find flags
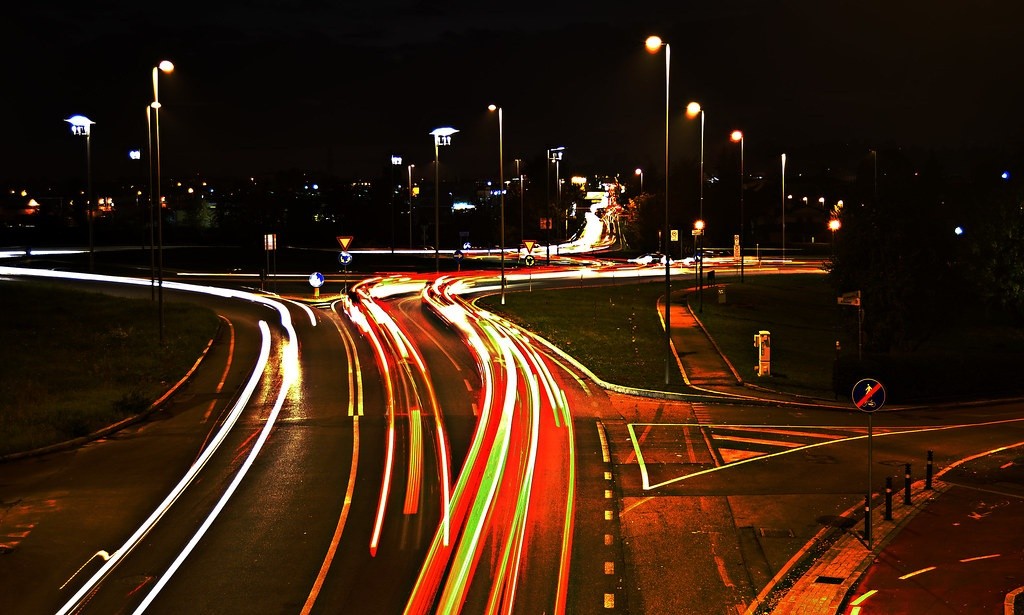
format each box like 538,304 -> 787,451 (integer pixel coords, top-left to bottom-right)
838,290 -> 859,306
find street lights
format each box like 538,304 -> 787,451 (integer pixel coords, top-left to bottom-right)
152,60 -> 174,347
62,114 -> 96,272
686,101 -> 704,284
644,34 -> 670,340
428,127 -> 461,274
781,151 -> 786,263
729,130 -> 745,282
635,168 -> 643,192
146,101 -> 162,302
487,104 -> 505,305
408,164 -> 416,249
